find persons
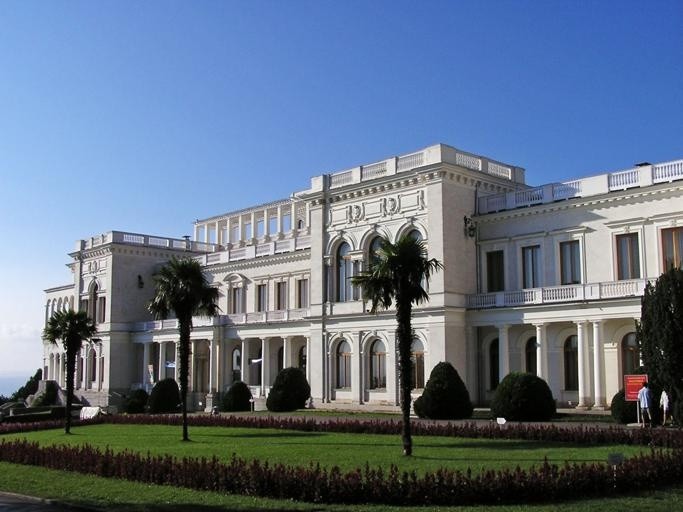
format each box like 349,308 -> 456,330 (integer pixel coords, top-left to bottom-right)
659,385 -> 674,427
637,381 -> 652,428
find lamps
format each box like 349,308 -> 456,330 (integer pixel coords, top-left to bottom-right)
464,216 -> 476,237
137,275 -> 144,289
